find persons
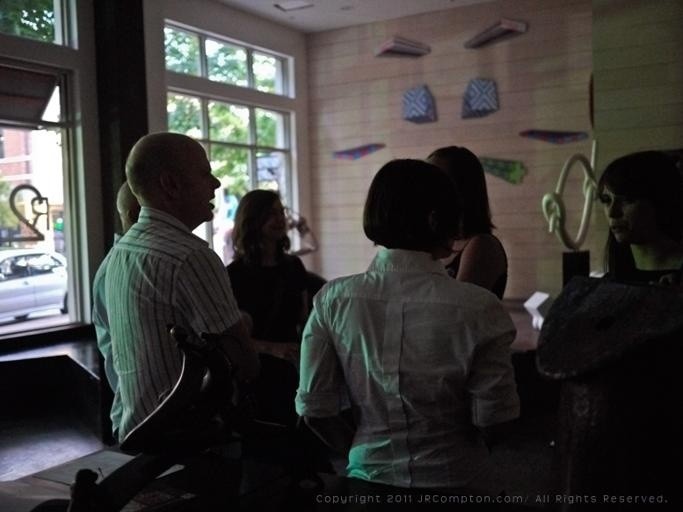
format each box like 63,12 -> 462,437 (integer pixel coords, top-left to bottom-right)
293,161 -> 521,512
92,180 -> 140,445
427,146 -> 508,301
541,151 -> 682,512
225,190 -> 309,361
91,133 -> 259,448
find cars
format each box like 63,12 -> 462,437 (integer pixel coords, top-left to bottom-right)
0,248 -> 68,321
